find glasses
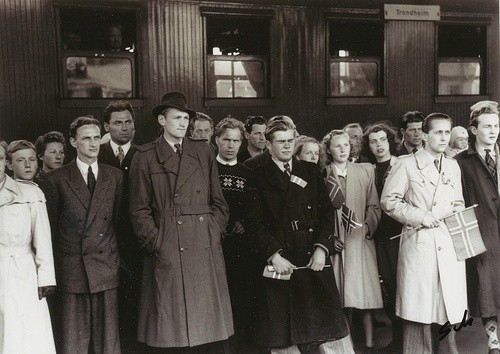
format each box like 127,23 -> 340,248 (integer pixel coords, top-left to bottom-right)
219,136 -> 241,145
350,135 -> 363,141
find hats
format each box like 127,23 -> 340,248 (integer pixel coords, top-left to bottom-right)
153,92 -> 196,119
469,100 -> 498,111
450,125 -> 469,148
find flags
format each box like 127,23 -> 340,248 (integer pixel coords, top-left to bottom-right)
327,169 -> 344,208
440,204 -> 487,262
263,265 -> 293,280
341,203 -> 364,235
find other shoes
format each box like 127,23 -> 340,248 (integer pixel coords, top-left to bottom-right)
365,344 -> 376,354
488,349 -> 500,354
379,341 -> 403,353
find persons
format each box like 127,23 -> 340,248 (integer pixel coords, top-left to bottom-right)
0,92 -> 500,354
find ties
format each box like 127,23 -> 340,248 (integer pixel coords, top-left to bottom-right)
412,148 -> 418,154
88,166 -> 97,197
117,146 -> 125,167
174,144 -> 182,160
484,149 -> 498,191
284,163 -> 291,178
434,159 -> 439,174
